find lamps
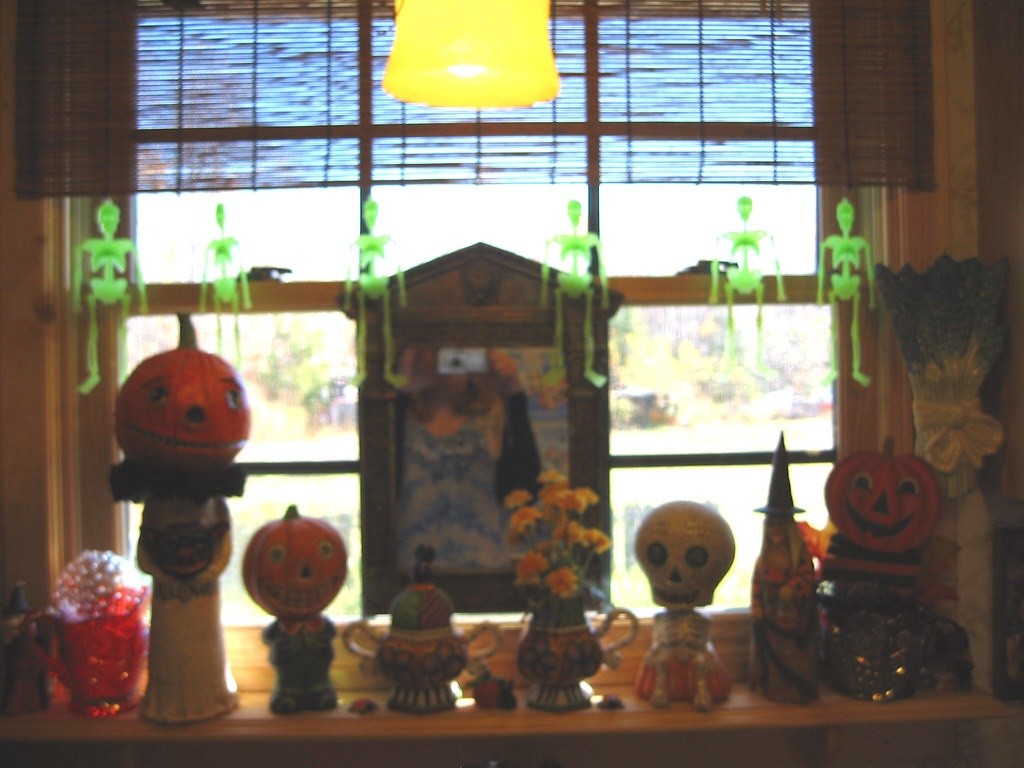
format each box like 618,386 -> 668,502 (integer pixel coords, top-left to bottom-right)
381,0 -> 561,109
